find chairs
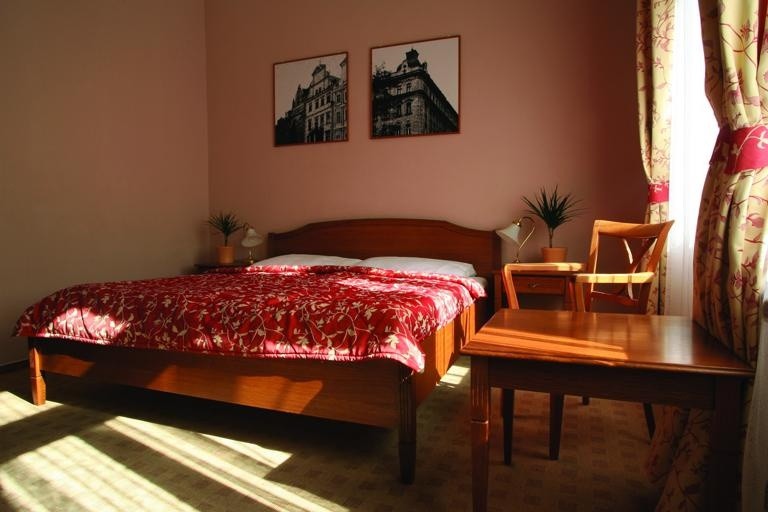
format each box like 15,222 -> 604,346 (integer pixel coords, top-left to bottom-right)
501,216 -> 680,471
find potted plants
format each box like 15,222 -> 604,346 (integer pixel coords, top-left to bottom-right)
201,212 -> 245,263
518,184 -> 586,261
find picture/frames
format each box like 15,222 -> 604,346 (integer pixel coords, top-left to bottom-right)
273,48 -> 350,149
369,34 -> 463,141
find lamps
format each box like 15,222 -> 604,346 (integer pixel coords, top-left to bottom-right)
491,213 -> 537,262
238,218 -> 263,261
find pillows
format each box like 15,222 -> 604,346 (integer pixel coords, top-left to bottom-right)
353,255 -> 478,282
247,253 -> 362,271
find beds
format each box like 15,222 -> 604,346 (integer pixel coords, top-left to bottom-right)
9,216 -> 506,484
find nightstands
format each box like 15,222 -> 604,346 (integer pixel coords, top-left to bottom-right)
488,265 -> 574,311
194,260 -> 252,273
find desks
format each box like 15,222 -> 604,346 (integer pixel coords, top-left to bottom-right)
455,307 -> 756,509
